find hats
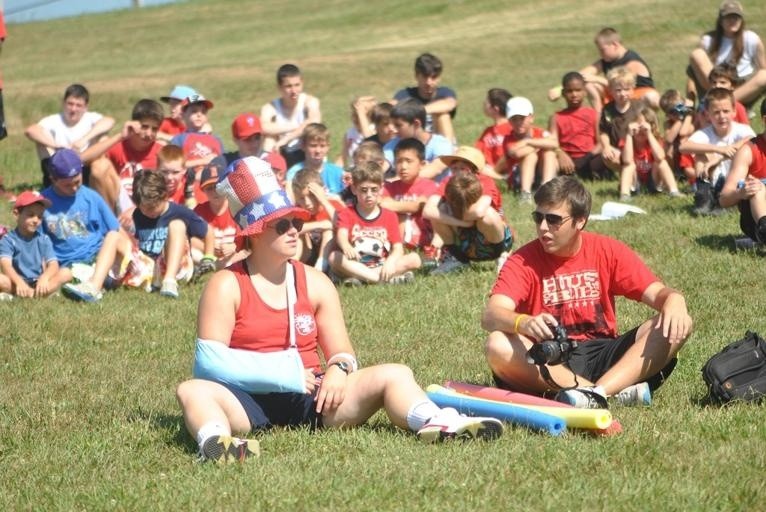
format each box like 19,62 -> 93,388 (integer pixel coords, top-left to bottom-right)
263,154 -> 286,172
201,165 -> 222,188
719,0 -> 744,17
180,94 -> 213,113
49,150 -> 81,178
232,112 -> 262,139
15,190 -> 49,208
505,96 -> 533,119
215,157 -> 310,253
160,85 -> 196,103
439,145 -> 485,171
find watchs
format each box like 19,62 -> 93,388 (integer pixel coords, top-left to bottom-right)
330,356 -> 354,373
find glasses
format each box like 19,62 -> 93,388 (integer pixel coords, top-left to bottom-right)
531,211 -> 570,226
262,219 -> 304,238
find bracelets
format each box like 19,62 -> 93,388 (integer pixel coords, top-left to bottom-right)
511,310 -> 525,334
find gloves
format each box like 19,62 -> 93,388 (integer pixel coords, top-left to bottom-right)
197,258 -> 216,275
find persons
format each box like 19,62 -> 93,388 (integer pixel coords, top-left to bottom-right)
719,100 -> 766,257
474,89 -> 561,205
175,155 -> 502,464
688,0 -> 765,111
390,53 -> 460,143
0,64 -> 517,301
547,28 -> 758,216
479,175 -> 692,410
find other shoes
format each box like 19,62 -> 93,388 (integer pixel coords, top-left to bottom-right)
2,292 -> 14,301
61,281 -> 105,303
390,271 -> 415,284
430,257 -> 470,276
345,277 -> 362,286
48,292 -> 62,299
159,281 -> 179,297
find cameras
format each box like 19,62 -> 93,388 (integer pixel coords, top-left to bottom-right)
524,324 -> 579,366
672,102 -> 690,118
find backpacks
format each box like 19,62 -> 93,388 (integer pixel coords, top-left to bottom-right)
700,329 -> 766,405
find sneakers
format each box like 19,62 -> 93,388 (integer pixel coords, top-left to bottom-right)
558,387 -> 609,408
416,407 -> 504,445
691,173 -> 753,252
614,381 -> 650,408
200,435 -> 260,463
